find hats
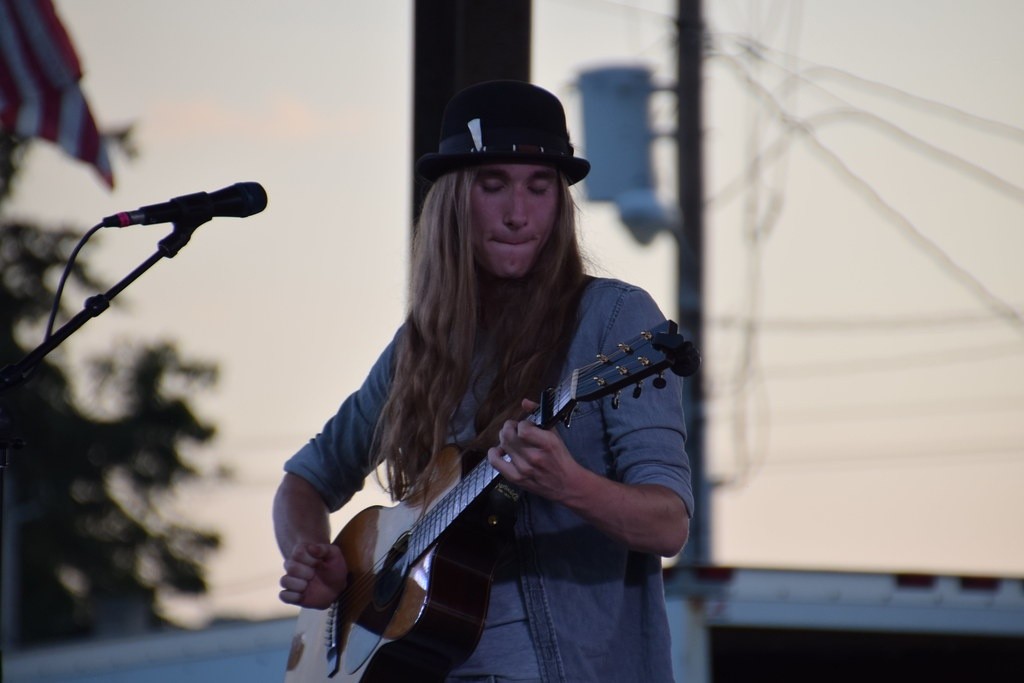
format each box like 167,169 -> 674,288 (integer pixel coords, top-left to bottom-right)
414,79 -> 591,187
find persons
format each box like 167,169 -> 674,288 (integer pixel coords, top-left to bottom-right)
267,81 -> 697,683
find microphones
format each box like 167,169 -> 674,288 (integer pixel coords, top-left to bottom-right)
104,181 -> 267,228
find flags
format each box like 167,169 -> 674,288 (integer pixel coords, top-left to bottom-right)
0,0 -> 119,189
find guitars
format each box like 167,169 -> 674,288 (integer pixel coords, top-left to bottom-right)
282,320 -> 703,683
